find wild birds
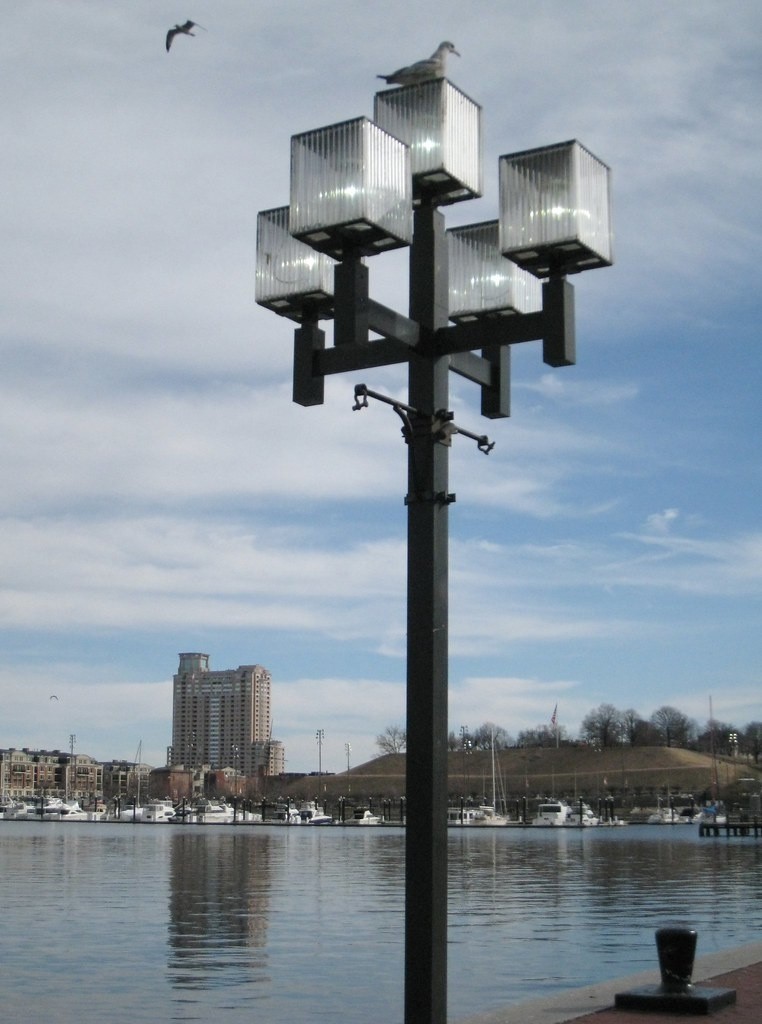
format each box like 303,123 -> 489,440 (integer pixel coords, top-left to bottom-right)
166,20 -> 208,53
49,696 -> 59,700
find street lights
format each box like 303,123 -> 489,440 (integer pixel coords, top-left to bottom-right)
257,77 -> 616,1024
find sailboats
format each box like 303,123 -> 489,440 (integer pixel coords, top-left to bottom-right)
690,695 -> 726,824
469,731 -> 510,827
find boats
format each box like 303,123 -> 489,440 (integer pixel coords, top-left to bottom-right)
448,808 -> 483,825
532,800 -> 600,826
344,808 -> 381,825
0,739 -> 332,826
648,807 -> 680,822
